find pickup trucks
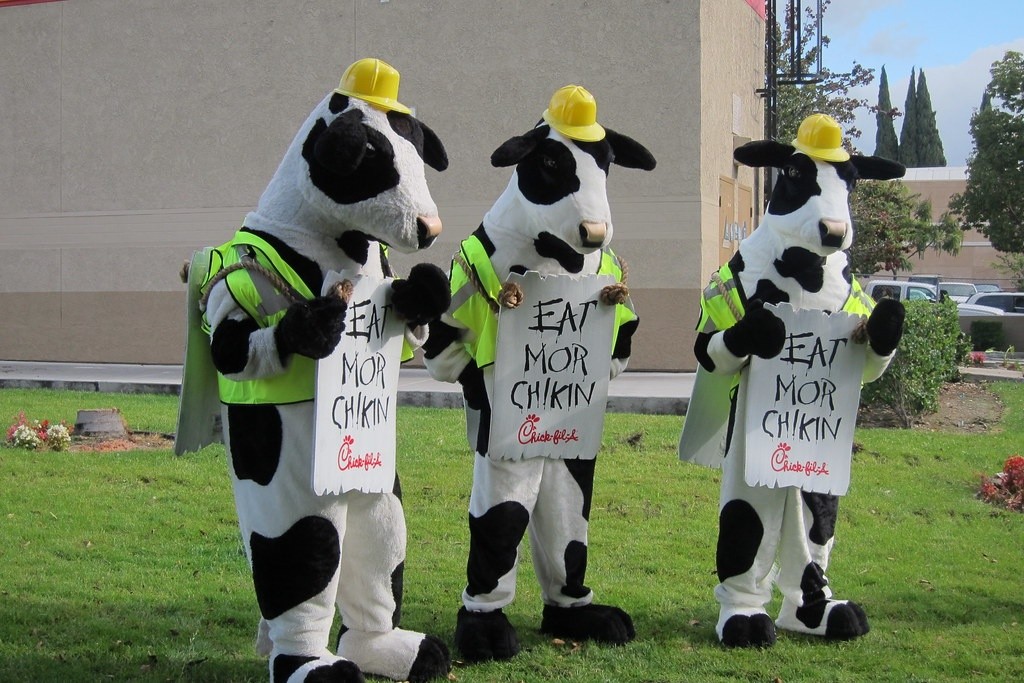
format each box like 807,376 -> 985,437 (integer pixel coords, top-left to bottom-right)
866,281 -> 1003,318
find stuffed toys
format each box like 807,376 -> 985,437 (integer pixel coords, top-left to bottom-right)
201,56 -> 453,682
694,111 -> 906,648
419,82 -> 658,660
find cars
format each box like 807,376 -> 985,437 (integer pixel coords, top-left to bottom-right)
974,283 -> 1003,295
938,282 -> 976,304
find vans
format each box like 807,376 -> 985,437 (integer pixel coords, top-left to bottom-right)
965,292 -> 1024,313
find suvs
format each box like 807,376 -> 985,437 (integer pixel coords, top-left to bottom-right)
909,273 -> 945,287
